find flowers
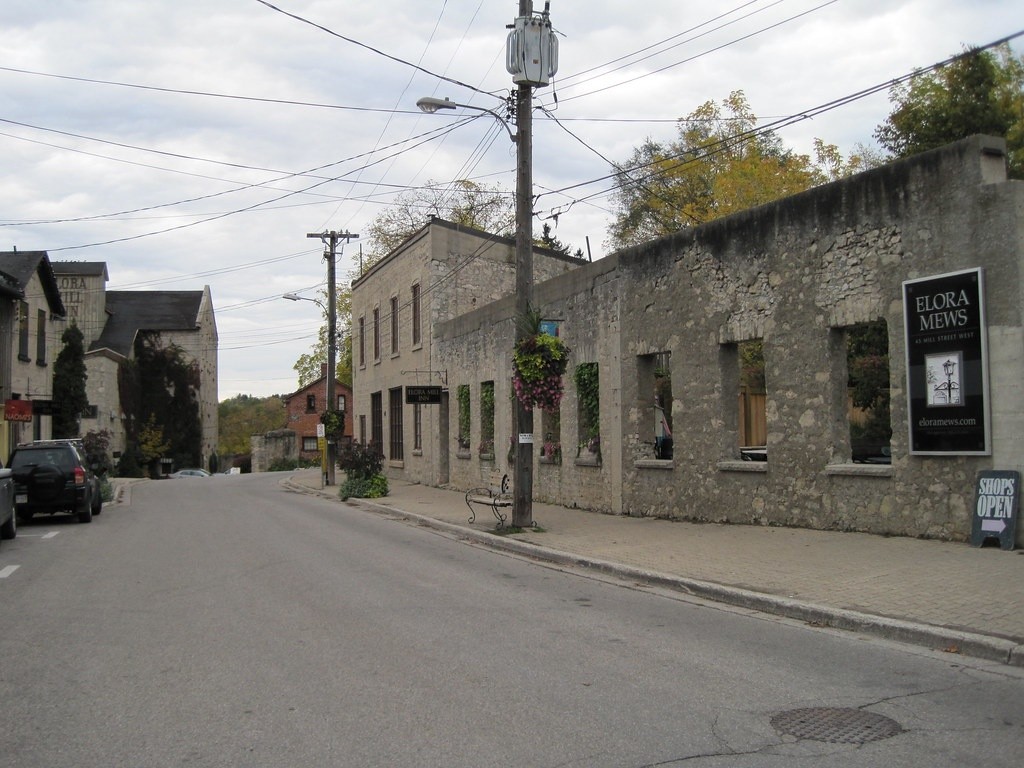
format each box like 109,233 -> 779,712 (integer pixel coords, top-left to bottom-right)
513,372 -> 563,410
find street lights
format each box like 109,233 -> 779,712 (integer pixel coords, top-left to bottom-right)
943,358 -> 957,404
417,96 -> 536,527
283,293 -> 337,487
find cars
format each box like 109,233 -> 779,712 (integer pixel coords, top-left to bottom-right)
172,468 -> 212,477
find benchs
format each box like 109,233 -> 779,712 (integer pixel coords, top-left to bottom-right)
465,468 -> 513,530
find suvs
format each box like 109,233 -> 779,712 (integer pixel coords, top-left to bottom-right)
5,439 -> 101,523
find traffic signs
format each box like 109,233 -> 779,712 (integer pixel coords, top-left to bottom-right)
971,469 -> 1021,551
